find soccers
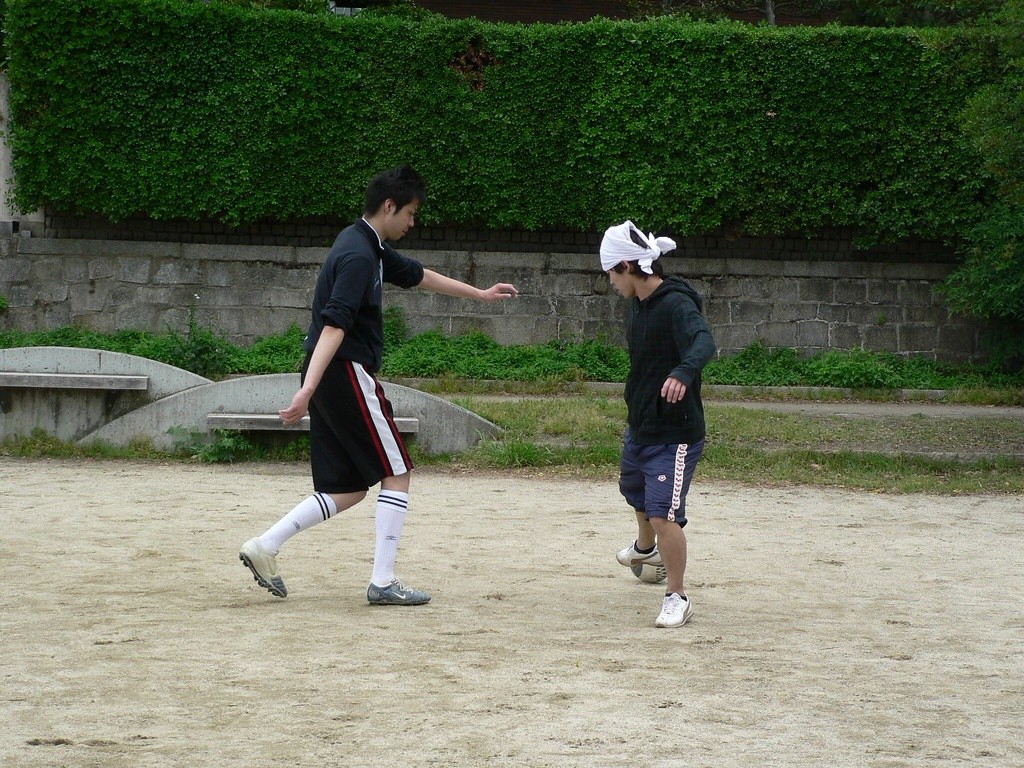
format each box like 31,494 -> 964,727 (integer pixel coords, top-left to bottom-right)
631,544 -> 667,583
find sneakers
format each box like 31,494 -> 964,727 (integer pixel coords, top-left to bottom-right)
616,538 -> 663,567
238,537 -> 287,598
366,575 -> 431,605
654,592 -> 695,628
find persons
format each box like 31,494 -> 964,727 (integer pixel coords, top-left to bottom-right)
239,160 -> 518,606
600,220 -> 717,629
